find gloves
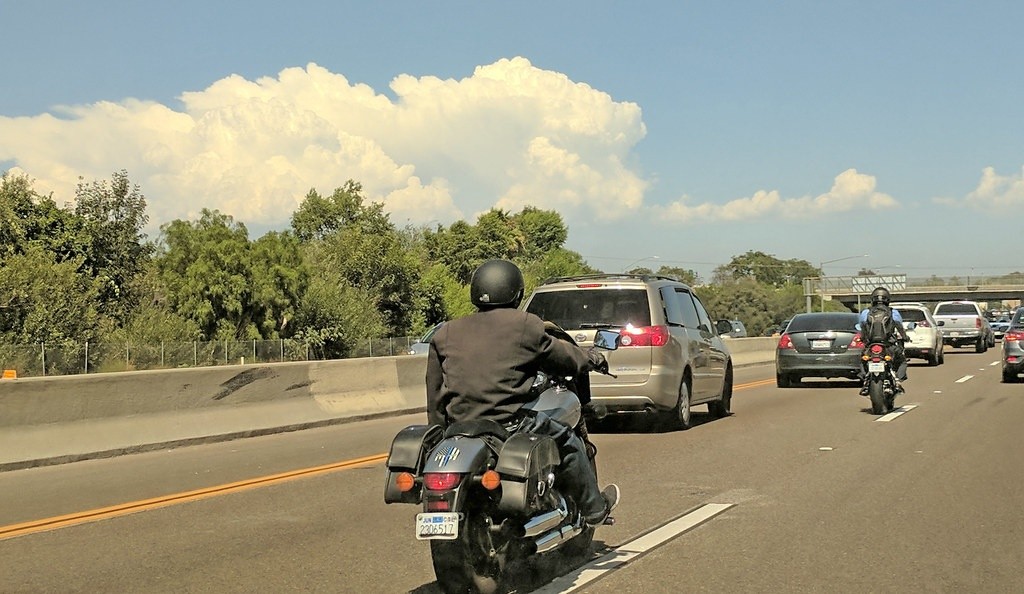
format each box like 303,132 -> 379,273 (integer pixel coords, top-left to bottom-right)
587,350 -> 608,375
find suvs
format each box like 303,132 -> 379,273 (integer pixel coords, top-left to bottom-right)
931,301 -> 996,353
889,301 -> 946,366
518,270 -> 735,429
986,306 -> 1024,382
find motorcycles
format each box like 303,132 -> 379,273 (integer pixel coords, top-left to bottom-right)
384,319 -> 622,594
854,323 -> 913,415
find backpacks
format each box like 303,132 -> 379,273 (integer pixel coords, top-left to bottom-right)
863,305 -> 896,341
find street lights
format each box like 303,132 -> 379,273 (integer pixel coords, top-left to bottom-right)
819,254 -> 870,313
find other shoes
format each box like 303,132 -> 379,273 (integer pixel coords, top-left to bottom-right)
896,383 -> 905,394
859,388 -> 868,395
585,484 -> 620,527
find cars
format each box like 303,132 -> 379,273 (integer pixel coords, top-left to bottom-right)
715,320 -> 748,339
402,319 -> 446,356
775,311 -> 867,388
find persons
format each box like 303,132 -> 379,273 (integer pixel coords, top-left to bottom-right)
426,258 -> 621,528
857,287 -> 910,396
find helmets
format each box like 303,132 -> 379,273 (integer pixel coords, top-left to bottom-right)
470,260 -> 525,308
870,287 -> 890,307
